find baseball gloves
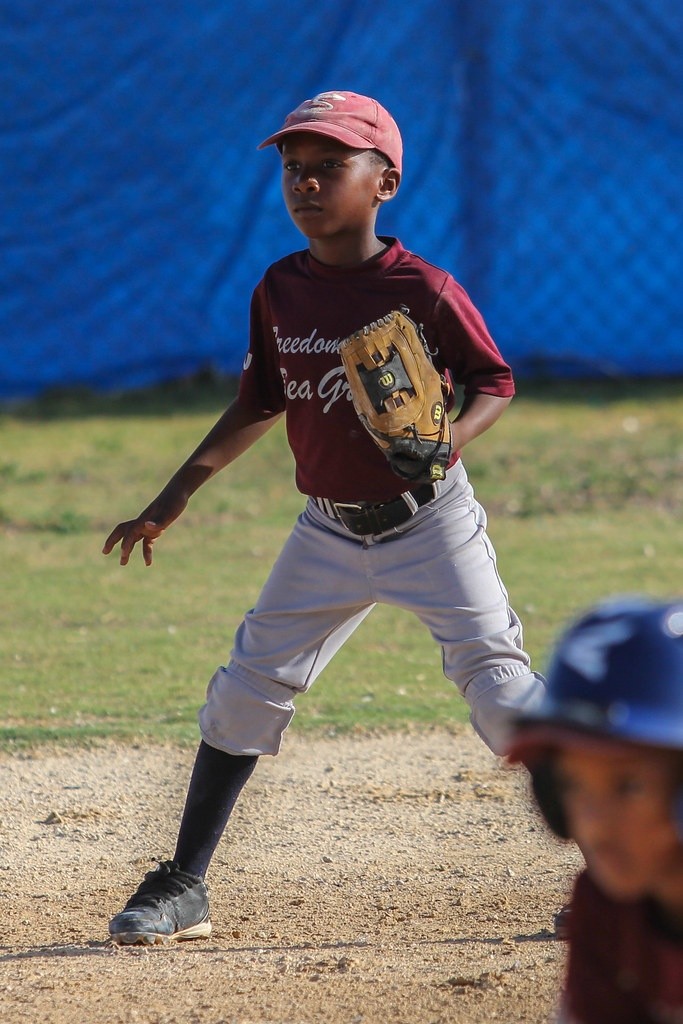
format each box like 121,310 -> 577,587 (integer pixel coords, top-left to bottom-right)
340,305 -> 450,484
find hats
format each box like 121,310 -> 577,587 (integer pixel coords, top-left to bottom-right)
258,91 -> 403,174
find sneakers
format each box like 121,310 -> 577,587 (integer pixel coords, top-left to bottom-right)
553,904 -> 571,937
109,857 -> 211,947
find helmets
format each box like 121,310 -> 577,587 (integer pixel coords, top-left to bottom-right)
504,600 -> 683,841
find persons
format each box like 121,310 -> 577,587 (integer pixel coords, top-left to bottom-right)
507,599 -> 683,1024
101,90 -> 542,948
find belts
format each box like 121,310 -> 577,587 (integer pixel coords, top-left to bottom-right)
308,484 -> 433,536
